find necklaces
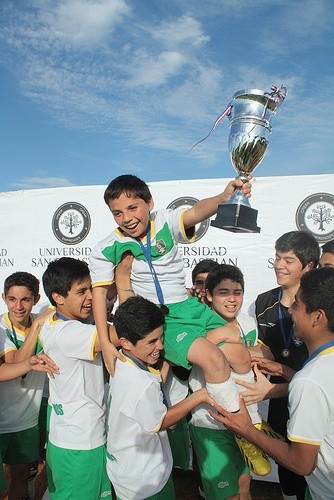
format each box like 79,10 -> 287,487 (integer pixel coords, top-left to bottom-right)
303,340 -> 334,368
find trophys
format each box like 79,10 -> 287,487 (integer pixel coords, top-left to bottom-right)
210,84 -> 288,233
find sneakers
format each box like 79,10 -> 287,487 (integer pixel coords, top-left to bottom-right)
235,434 -> 272,476
254,418 -> 286,441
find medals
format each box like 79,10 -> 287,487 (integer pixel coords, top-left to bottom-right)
20,379 -> 26,386
281,348 -> 290,357
160,304 -> 169,315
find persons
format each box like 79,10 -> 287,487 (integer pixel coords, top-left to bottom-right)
38,257 -> 122,500
161,232 -> 334,500
88,174 -> 285,476
105,294 -> 218,500
0,272 -> 56,500
